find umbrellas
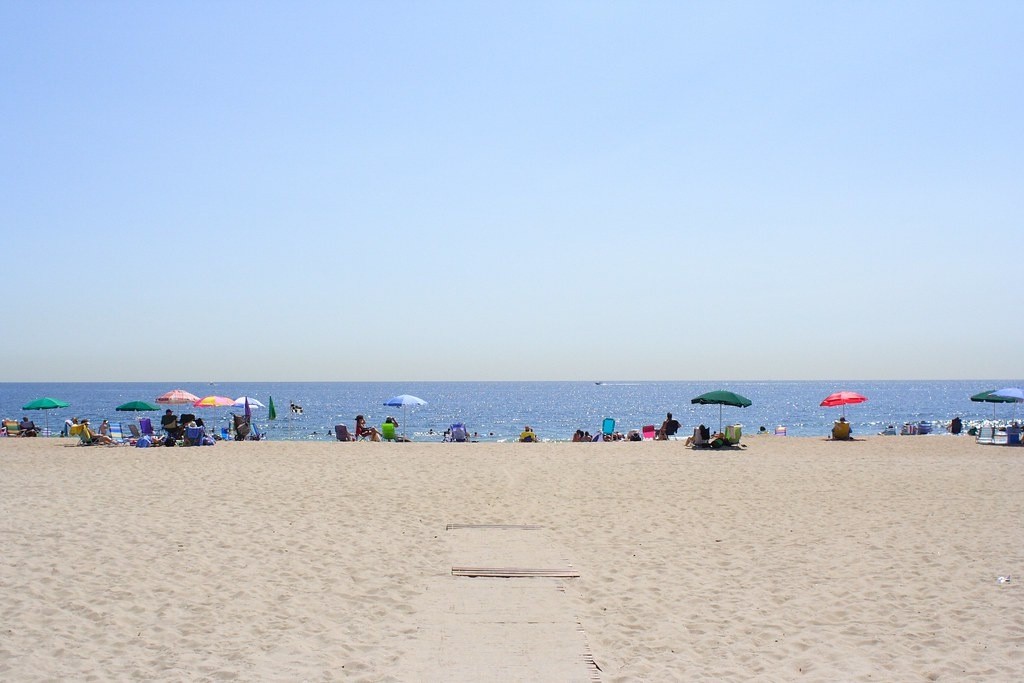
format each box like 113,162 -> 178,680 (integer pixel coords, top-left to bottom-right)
383,394 -> 429,442
22,396 -> 69,437
691,389 -> 752,432
269,395 -> 276,420
970,389 -> 1017,426
991,388 -> 1024,427
820,391 -> 869,416
156,390 -> 266,432
116,400 -> 161,429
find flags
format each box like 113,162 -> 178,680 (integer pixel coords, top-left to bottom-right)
291,401 -> 304,413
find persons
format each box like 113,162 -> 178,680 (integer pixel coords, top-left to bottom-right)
831,417 -> 852,433
60,417 -> 119,445
430,422 -> 478,442
877,417 -> 960,436
161,409 -> 206,440
573,412 -> 705,446
998,422 -> 1024,447
336,415 -> 398,442
520,426 -> 538,443
21,416 -> 42,433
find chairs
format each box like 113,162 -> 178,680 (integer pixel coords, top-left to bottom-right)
948,418 -> 963,436
355,427 -> 372,442
381,422 -> 399,442
774,426 -> 787,436
0,426 -> 8,437
335,425 -> 354,441
885,421 -> 932,436
660,420 -> 679,441
976,425 -> 1023,446
449,423 -> 468,442
832,420 -> 850,440
598,418 -> 615,436
5,419 -> 27,438
64,415 -> 268,447
19,421 -> 42,437
689,426 -> 710,446
724,425 -> 742,447
519,432 -> 535,443
641,425 -> 656,441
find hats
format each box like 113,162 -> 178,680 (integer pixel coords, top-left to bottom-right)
165,409 -> 173,413
